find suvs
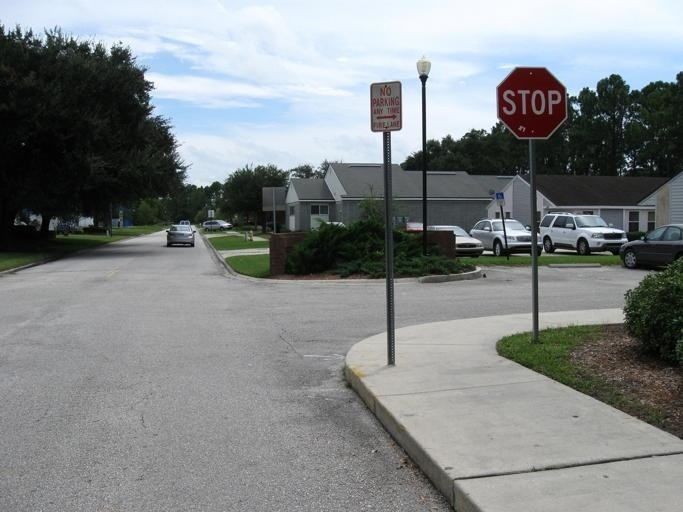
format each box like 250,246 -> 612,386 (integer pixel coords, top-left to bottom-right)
539,210 -> 628,255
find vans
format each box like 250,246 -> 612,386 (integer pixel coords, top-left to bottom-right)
202,220 -> 233,232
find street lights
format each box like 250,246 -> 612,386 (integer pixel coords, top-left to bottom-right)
415,55 -> 432,255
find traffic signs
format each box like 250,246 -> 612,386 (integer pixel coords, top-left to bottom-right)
370,82 -> 402,132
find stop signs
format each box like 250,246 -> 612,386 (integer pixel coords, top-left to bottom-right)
496,66 -> 569,141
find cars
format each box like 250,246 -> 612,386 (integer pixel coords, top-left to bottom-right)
165,220 -> 196,248
469,219 -> 542,258
619,224 -> 682,269
426,225 -> 484,257
